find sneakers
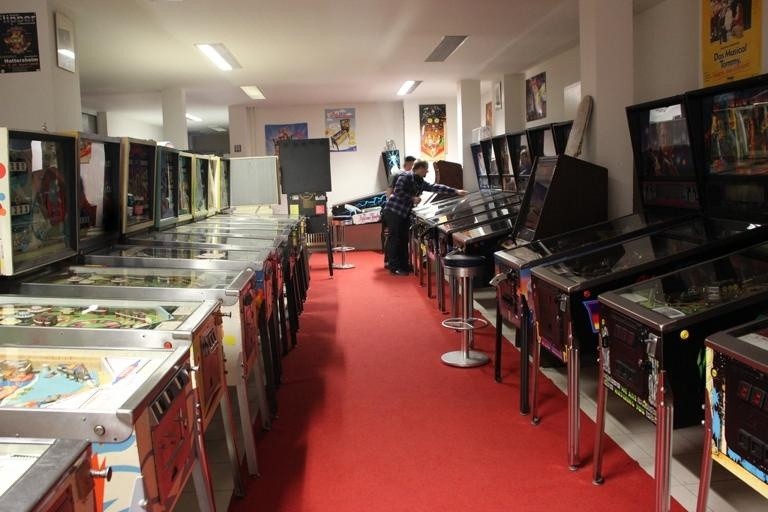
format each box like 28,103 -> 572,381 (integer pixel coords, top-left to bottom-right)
385,261 -> 411,275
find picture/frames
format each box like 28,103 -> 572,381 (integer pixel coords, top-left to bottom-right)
698,0 -> 768,90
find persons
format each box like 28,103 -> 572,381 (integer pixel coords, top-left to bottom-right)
384,156 -> 416,269
382,159 -> 467,276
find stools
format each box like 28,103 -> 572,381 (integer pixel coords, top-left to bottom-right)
331,215 -> 355,269
441,254 -> 490,367
